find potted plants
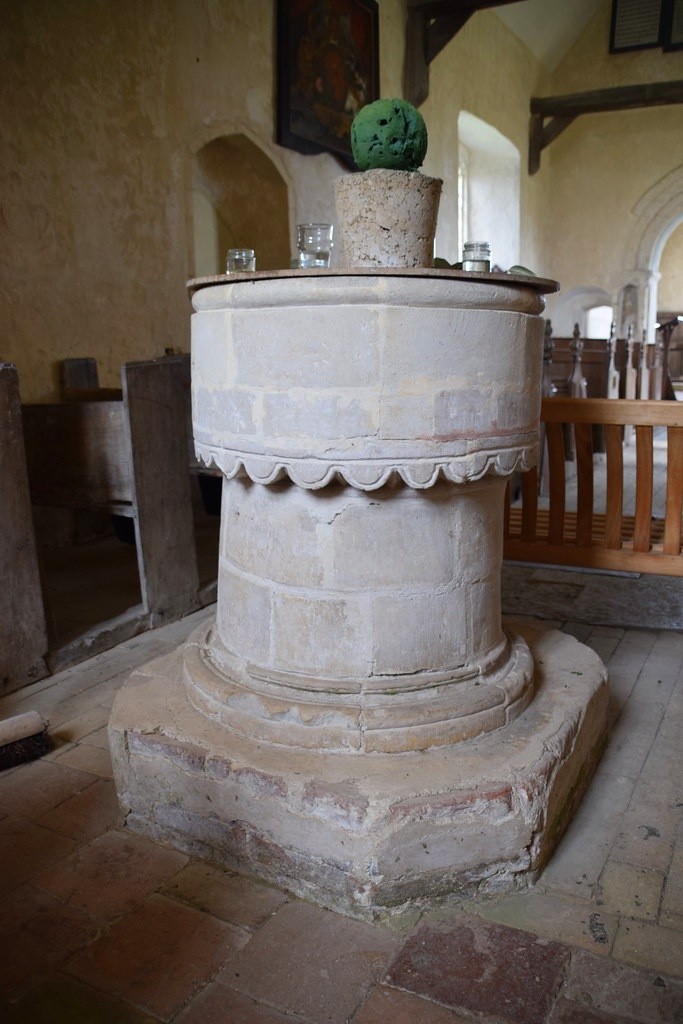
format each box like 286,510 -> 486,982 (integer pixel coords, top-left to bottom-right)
335,97 -> 444,266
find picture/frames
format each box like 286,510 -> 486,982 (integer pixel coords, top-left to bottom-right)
276,0 -> 379,173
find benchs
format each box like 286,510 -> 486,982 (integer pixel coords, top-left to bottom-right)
0,355 -> 222,697
503,318 -> 683,577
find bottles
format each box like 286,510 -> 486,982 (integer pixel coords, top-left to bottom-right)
164,347 -> 175,356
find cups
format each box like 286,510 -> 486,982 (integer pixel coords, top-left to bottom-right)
464,242 -> 488,254
226,248 -> 255,275
297,223 -> 334,269
231,256 -> 256,273
462,250 -> 491,272
